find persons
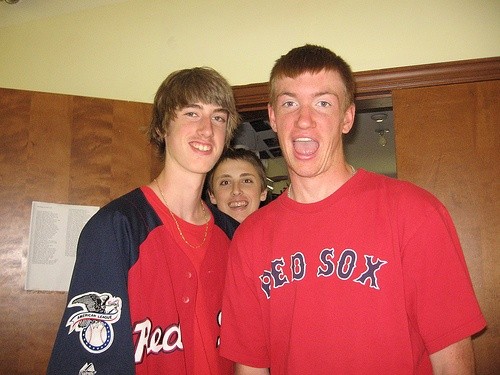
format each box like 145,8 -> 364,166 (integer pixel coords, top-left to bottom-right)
46,67 -> 240,375
205,149 -> 275,224
220,44 -> 487,375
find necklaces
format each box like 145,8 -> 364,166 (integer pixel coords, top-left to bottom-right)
154,172 -> 208,249
